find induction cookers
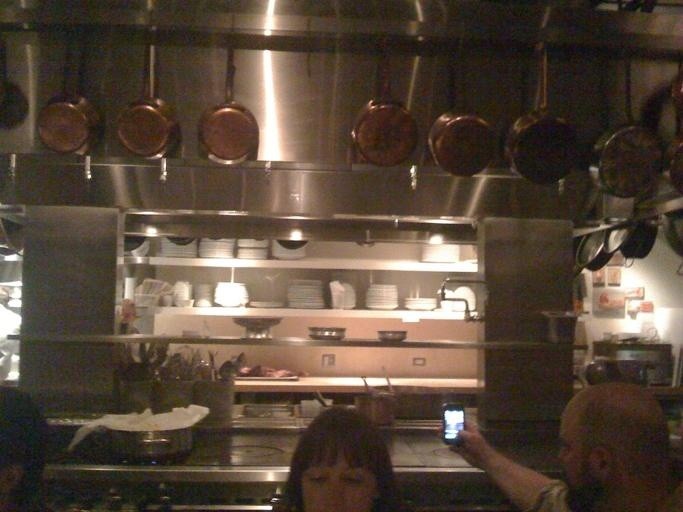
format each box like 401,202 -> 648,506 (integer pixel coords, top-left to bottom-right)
36,432 -> 509,510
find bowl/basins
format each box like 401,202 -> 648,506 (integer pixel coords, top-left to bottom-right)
376,330 -> 406,341
354,396 -> 395,424
307,325 -> 346,339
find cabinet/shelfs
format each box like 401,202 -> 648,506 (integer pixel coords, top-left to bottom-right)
109,210 -> 488,320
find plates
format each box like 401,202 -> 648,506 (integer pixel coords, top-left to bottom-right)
121,277 -> 475,312
120,230 -> 307,263
233,399 -> 333,416
421,244 -> 460,264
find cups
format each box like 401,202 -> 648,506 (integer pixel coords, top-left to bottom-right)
126,380 -> 236,422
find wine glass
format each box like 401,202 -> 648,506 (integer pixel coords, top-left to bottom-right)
234,317 -> 280,339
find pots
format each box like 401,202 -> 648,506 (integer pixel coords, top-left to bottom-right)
115,21 -> 177,160
351,37 -> 683,199
195,29 -> 258,163
96,423 -> 192,458
37,30 -> 105,155
572,209 -> 683,281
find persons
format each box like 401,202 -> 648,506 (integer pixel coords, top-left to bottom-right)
435,382 -> 683,512
0,386 -> 49,511
287,405 -> 401,511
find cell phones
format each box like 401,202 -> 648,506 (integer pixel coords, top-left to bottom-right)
441,402 -> 467,445
